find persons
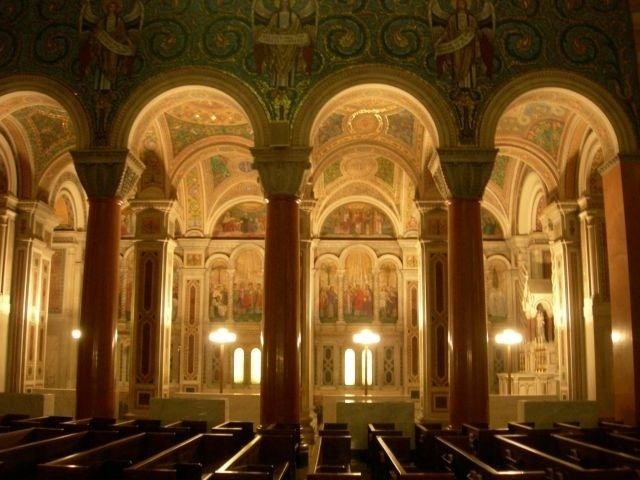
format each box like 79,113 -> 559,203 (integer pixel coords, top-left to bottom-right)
331,207 -> 386,235
221,211 -> 265,233
318,283 -> 398,323
210,280 -> 264,323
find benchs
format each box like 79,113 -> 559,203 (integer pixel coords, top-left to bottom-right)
0,415 -> 640,480
